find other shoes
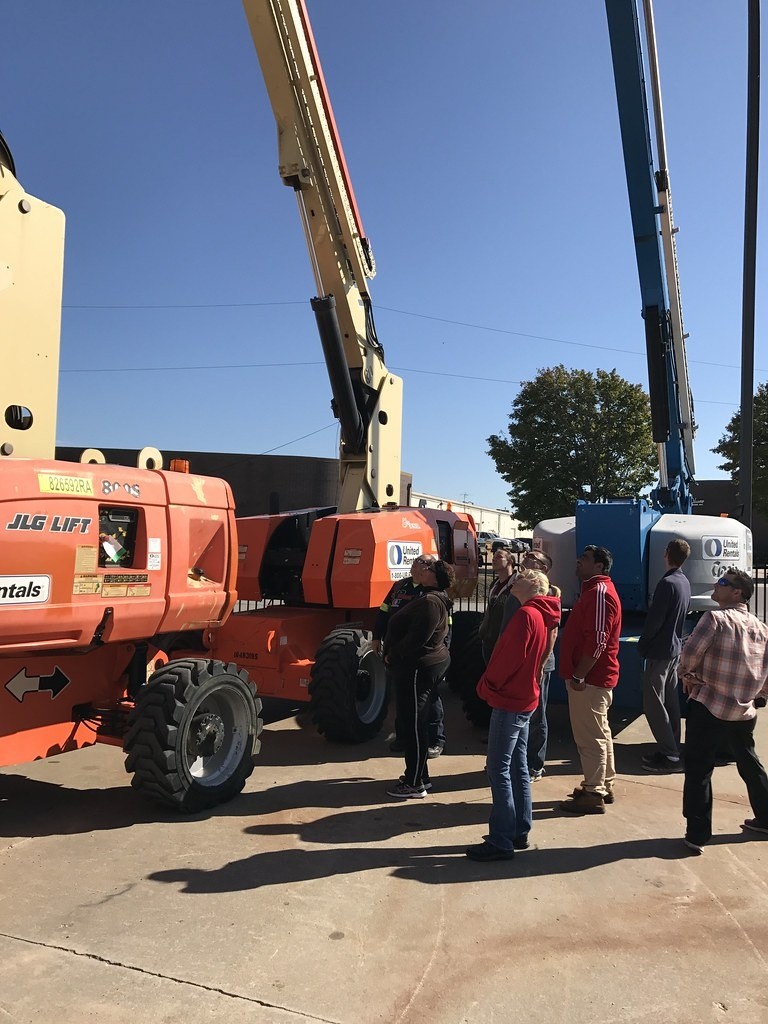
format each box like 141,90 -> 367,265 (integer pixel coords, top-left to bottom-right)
482,835 -> 529,849
641,757 -> 684,772
390,740 -> 402,750
643,753 -> 663,762
559,787 -> 604,815
568,786 -> 614,804
427,745 -> 443,758
466,842 -> 516,862
480,736 -> 488,744
485,766 -> 487,771
530,774 -> 542,783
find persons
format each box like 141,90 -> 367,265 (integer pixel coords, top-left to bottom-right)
373,553 -> 453,758
677,567 -> 768,852
476,549 -> 519,772
558,543 -> 622,814
494,548 -> 561,783
385,560 -> 455,798
465,569 -> 562,862
636,540 -> 693,772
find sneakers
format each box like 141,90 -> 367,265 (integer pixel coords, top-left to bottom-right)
384,781 -> 428,797
685,837 -> 701,848
745,818 -> 768,833
399,776 -> 432,790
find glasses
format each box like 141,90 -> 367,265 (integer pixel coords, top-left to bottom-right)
515,575 -> 534,581
718,578 -> 744,595
522,554 -> 545,565
416,558 -> 432,565
427,566 -> 436,573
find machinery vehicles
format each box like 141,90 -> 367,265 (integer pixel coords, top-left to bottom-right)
461,1 -> 755,729
3,0 -> 477,809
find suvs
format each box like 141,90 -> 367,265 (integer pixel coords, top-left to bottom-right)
476,531 -> 512,552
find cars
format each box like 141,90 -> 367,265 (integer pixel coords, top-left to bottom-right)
503,538 -> 531,554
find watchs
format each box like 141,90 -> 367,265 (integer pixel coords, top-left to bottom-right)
571,674 -> 585,684
